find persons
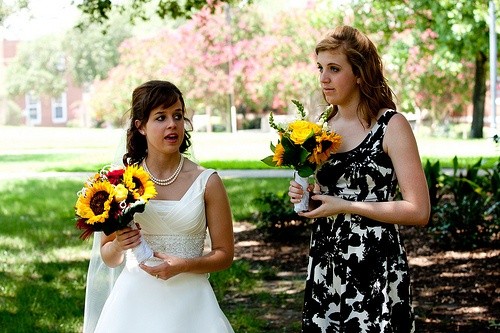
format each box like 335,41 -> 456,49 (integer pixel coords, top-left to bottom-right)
82,79 -> 235,333
288,26 -> 431,333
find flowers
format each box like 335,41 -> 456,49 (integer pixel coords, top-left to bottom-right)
74,161 -> 154,264
259,99 -> 343,213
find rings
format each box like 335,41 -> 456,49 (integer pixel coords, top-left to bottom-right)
156,275 -> 158,278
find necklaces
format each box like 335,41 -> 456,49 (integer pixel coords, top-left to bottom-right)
142,154 -> 184,186
